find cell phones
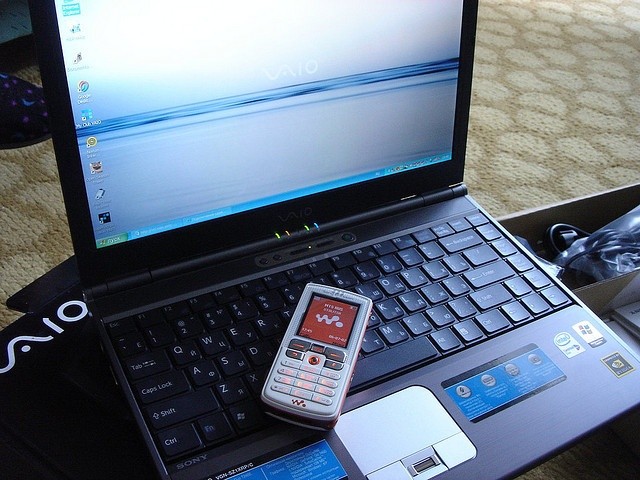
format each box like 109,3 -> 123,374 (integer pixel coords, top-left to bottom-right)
256,281 -> 374,434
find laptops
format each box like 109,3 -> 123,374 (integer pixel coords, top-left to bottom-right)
25,0 -> 640,480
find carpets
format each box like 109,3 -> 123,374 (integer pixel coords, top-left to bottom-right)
1,1 -> 640,326
